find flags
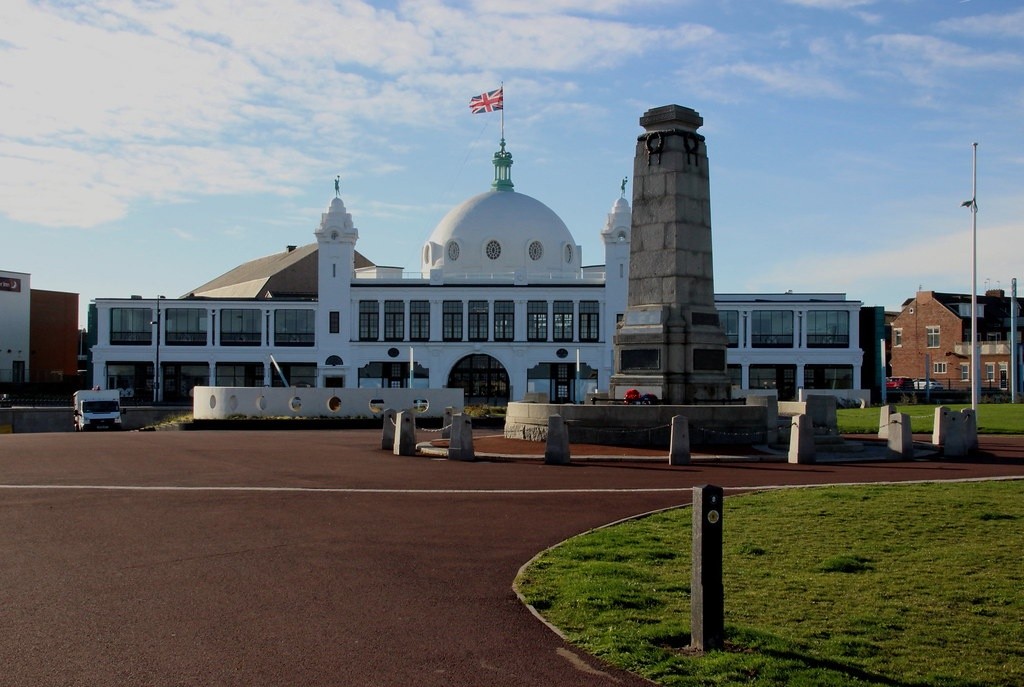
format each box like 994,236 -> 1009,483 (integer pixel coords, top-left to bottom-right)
468,87 -> 504,114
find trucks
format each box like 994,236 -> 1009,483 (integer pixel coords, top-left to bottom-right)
73,385 -> 125,431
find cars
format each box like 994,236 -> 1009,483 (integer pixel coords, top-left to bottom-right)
913,377 -> 944,391
886,376 -> 914,391
